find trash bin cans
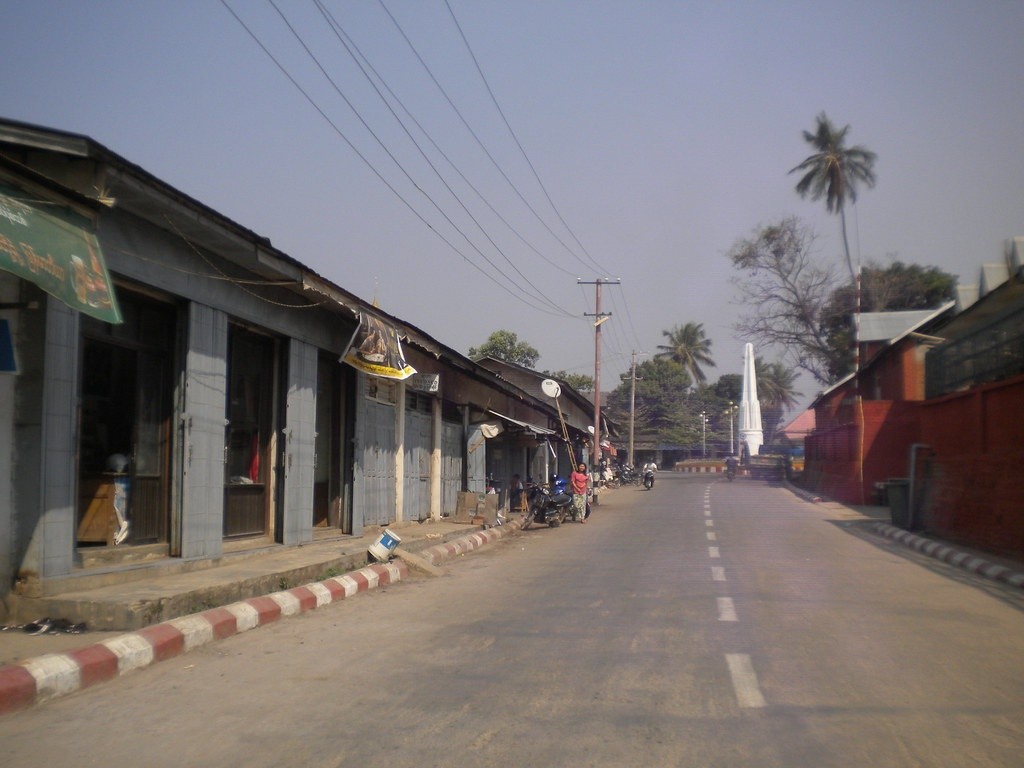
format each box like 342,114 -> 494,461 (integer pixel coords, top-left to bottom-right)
884,479 -> 911,530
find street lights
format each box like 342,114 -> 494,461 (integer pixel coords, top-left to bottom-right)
722,400 -> 739,454
700,410 -> 710,460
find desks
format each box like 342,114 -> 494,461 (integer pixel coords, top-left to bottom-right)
73,470 -> 128,547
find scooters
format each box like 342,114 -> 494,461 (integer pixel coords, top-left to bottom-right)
724,463 -> 738,481
613,462 -> 641,486
642,470 -> 656,490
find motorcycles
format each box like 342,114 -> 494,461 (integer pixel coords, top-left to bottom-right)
551,473 -> 591,519
521,481 -> 572,530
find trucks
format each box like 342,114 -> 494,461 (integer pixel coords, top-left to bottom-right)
749,442 -> 797,482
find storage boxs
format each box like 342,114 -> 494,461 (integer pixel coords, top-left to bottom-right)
473,515 -> 485,525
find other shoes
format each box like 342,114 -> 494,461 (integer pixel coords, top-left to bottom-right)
581,519 -> 585,523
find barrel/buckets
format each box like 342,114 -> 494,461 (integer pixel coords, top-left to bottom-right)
366,528 -> 402,562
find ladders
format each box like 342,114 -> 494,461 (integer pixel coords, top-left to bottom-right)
555,398 -> 579,472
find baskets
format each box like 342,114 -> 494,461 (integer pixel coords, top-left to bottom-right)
533,494 -> 552,510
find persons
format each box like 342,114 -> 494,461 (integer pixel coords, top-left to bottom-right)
642,458 -> 657,485
510,475 -> 525,507
358,328 -> 387,357
725,454 -> 737,476
571,463 -> 590,524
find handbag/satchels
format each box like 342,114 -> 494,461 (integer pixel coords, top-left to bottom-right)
585,502 -> 591,518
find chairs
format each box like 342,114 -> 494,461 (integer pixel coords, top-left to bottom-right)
510,489 -> 527,512
